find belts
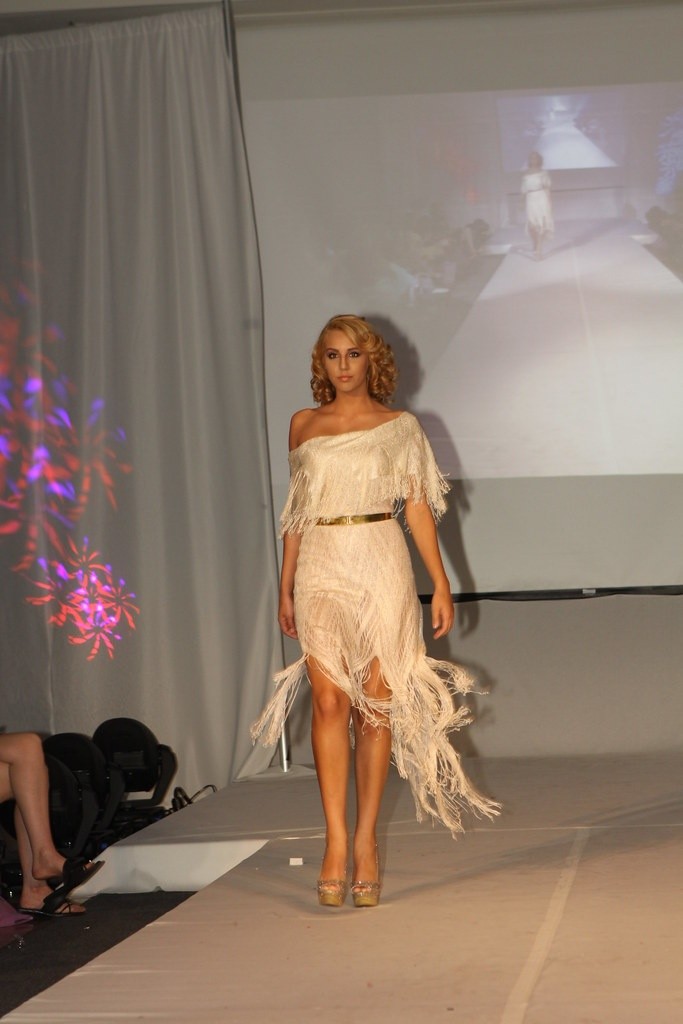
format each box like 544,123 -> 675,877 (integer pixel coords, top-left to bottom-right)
307,512 -> 396,526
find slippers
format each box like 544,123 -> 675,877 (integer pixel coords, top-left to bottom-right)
43,857 -> 104,903
18,890 -> 88,918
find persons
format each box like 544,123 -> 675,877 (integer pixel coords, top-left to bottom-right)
249,316 -> 503,908
521,149 -> 553,261
1,733 -> 105,923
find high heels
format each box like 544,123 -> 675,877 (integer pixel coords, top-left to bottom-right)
349,842 -> 378,906
319,854 -> 349,907
